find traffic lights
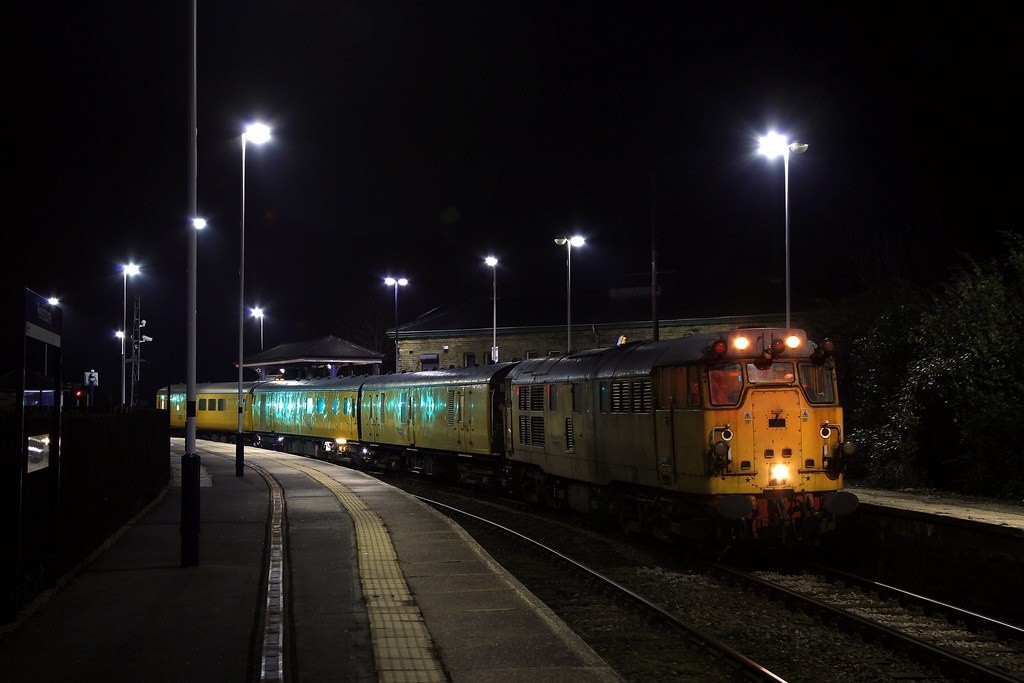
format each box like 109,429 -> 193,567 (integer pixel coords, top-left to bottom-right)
75,390 -> 82,398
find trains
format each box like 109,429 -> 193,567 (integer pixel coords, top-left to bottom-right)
155,327 -> 858,564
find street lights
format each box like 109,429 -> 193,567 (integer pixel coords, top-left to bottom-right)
759,133 -> 808,329
385,278 -> 408,375
485,256 -> 498,363
554,234 -> 584,355
44,297 -> 59,378
115,263 -> 140,415
235,122 -> 271,478
251,306 -> 263,350
